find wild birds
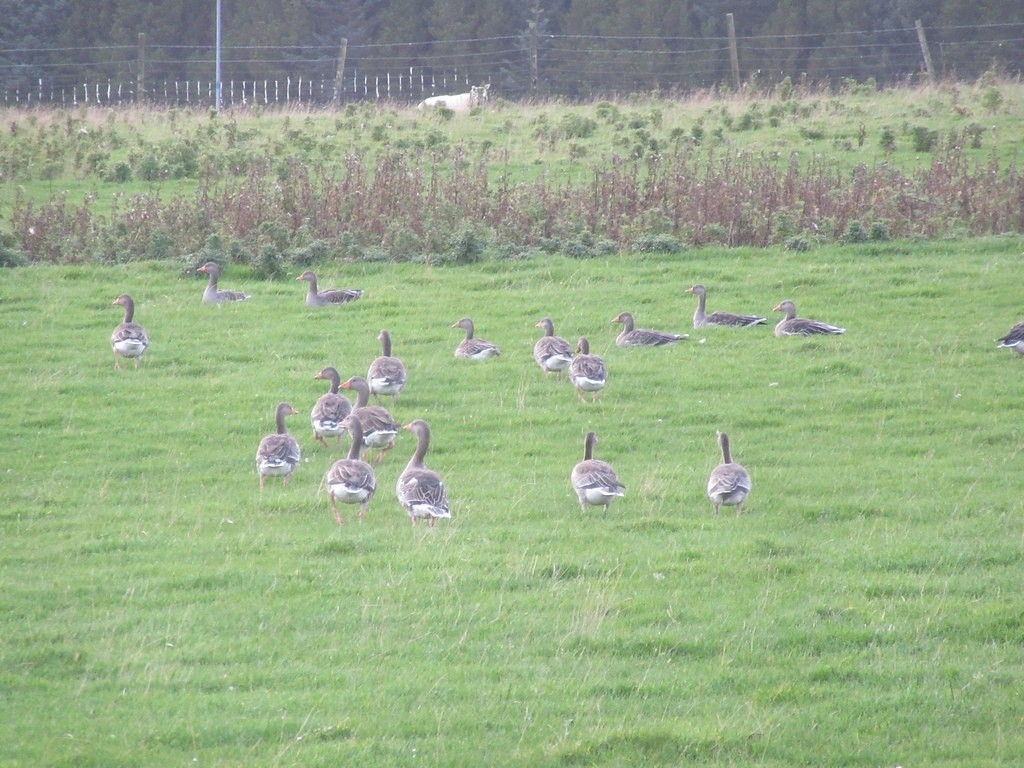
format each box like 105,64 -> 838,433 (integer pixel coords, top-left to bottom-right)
396,419 -> 452,529
197,261 -> 251,309
366,329 -> 407,408
110,294 -> 148,371
311,366 -> 352,449
995,320 -> 1024,358
772,300 -> 847,337
533,318 -> 575,381
571,431 -> 626,520
569,335 -> 608,404
326,414 -> 376,528
706,428 -> 753,522
452,317 -> 502,362
296,270 -> 365,308
610,312 -> 690,349
684,284 -> 768,329
256,403 -> 300,494
338,376 -> 401,465
